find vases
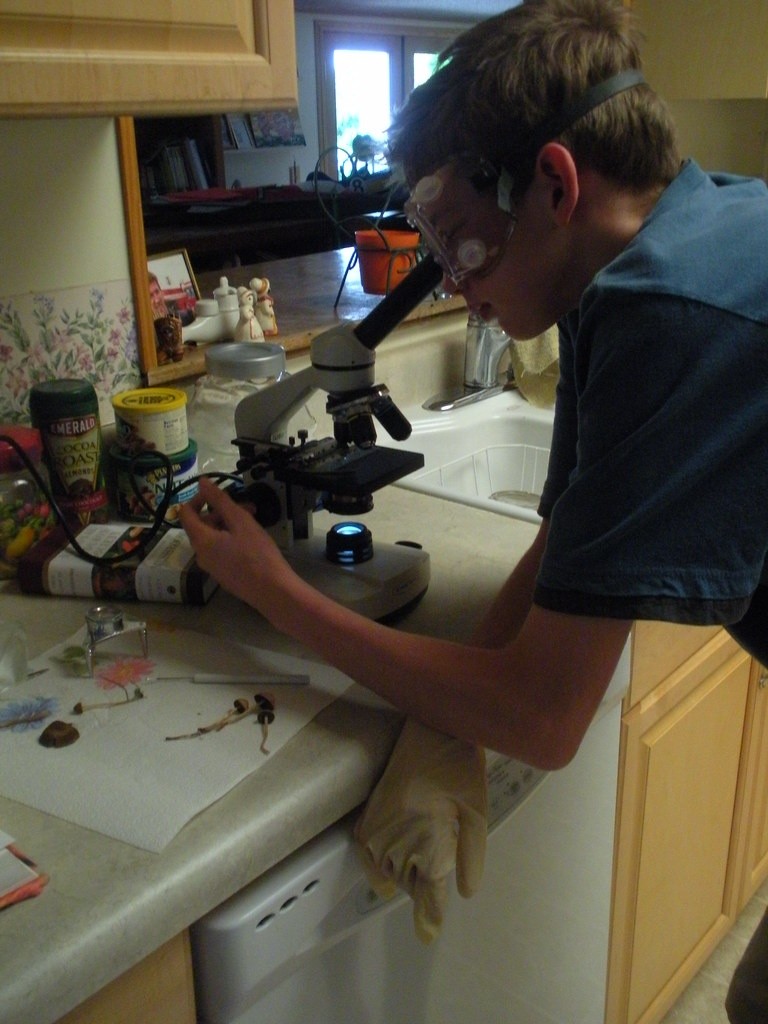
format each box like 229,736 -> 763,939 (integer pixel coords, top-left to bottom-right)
355,231 -> 420,296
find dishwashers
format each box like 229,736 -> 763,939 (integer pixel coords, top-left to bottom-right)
197,633 -> 632,1024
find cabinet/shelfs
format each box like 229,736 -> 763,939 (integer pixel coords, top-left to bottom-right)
0,0 -> 299,196
606,619 -> 768,1024
55,928 -> 197,1024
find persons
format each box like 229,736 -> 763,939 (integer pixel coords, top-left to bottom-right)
145,266 -> 196,365
175,0 -> 768,1023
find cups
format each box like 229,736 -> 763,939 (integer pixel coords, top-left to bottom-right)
355,230 -> 419,296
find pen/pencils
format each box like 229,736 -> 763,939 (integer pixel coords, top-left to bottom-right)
194,673 -> 311,684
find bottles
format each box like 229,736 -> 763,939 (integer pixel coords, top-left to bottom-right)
192,342 -> 316,482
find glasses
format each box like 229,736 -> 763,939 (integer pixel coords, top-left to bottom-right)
404,72 -> 646,291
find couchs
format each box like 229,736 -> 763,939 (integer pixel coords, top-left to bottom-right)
142,186 -> 409,273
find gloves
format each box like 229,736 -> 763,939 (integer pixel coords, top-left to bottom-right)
353,714 -> 488,947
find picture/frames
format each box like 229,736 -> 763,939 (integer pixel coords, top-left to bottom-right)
146,249 -> 202,329
228,119 -> 255,150
221,114 -> 236,152
